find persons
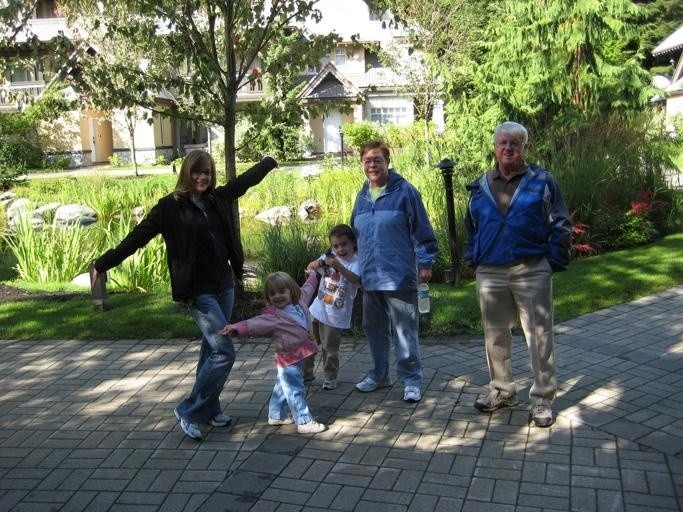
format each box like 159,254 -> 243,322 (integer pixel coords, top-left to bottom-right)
90,151 -> 280,443
300,225 -> 362,390
349,140 -> 440,405
217,269 -> 328,434
464,120 -> 572,427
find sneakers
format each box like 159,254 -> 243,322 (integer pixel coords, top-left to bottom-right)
530,405 -> 553,426
323,378 -> 336,390
268,416 -> 295,425
356,377 -> 391,392
474,391 -> 518,412
174,407 -> 231,441
404,386 -> 421,401
298,421 -> 325,433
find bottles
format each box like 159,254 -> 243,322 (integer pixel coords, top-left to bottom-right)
416,283 -> 430,314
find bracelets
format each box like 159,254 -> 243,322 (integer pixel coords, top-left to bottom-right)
317,258 -> 325,267
